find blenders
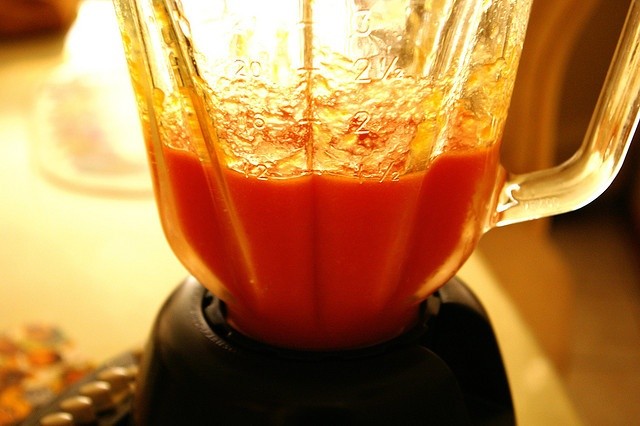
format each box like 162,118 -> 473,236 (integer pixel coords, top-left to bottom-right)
113,2 -> 640,425
